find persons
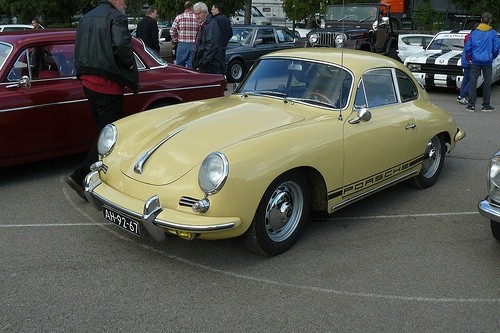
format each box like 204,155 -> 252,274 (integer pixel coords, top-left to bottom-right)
136,6 -> 160,55
192,2 -> 222,73
66,0 -> 139,202
211,3 -> 233,81
170,1 -> 199,68
457,23 -> 479,104
465,12 -> 500,112
21,44 -> 60,79
32,18 -> 43,29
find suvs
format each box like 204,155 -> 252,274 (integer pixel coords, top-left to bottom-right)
307,1 -> 407,62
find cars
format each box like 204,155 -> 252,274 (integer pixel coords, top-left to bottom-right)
0,24 -> 37,32
81,45 -> 469,259
289,15 -> 325,39
397,33 -> 435,63
405,27 -> 500,97
223,24 -> 306,83
130,26 -> 175,62
478,150 -> 500,239
127,16 -> 140,31
1,27 -> 234,170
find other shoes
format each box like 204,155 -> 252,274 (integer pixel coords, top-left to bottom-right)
482,106 -> 496,112
457,95 -> 469,105
465,105 -> 475,112
65,174 -> 88,202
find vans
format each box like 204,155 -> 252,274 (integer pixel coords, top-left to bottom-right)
231,5 -> 272,26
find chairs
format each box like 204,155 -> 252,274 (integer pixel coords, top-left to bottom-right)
38,54 -> 76,78
